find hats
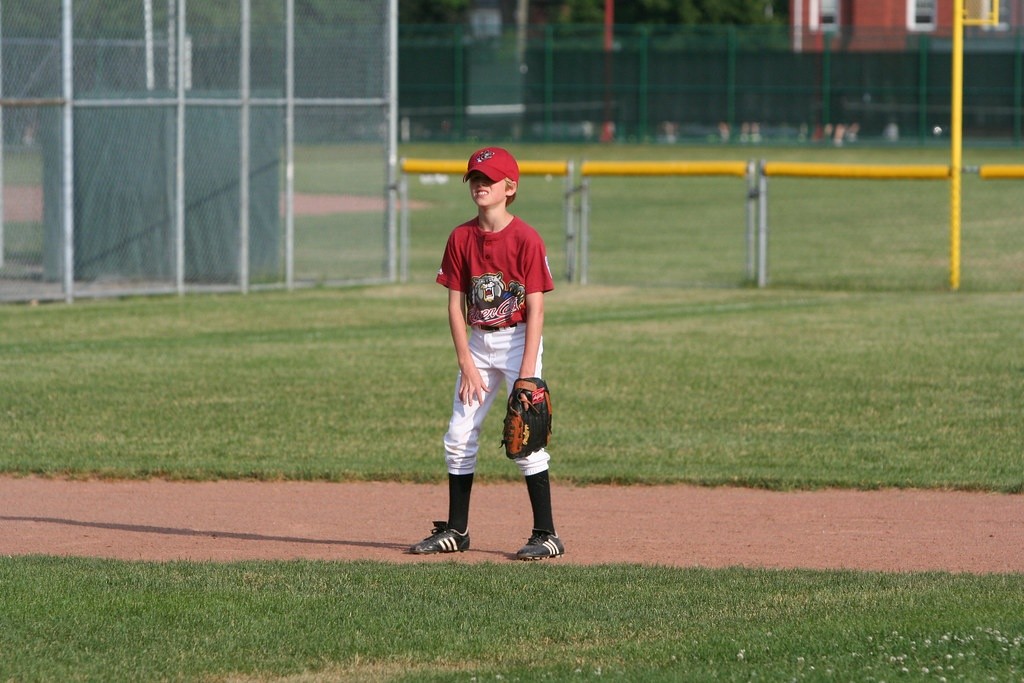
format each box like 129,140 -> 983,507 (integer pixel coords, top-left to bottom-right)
463,147 -> 518,185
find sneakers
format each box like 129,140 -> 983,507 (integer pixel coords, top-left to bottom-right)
410,521 -> 471,555
516,529 -> 564,561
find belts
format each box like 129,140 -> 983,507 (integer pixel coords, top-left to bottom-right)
480,322 -> 518,333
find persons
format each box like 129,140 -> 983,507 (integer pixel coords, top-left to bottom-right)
411,148 -> 565,560
581,119 -> 861,146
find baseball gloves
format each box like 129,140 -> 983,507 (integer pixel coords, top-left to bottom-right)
497,377 -> 553,461
